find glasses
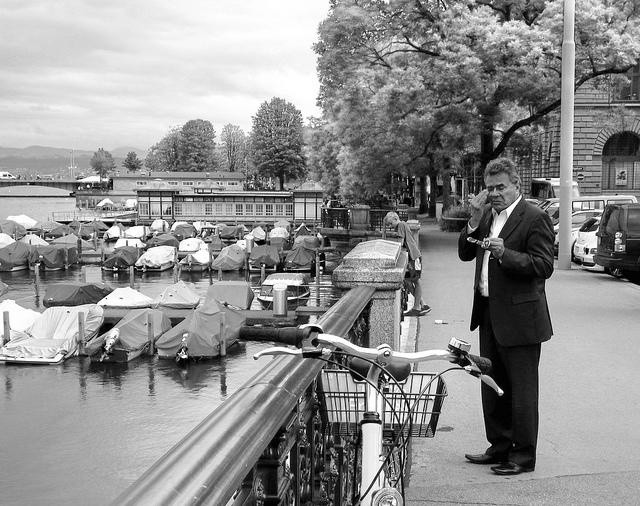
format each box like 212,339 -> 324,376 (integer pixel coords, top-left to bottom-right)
465,225 -> 487,246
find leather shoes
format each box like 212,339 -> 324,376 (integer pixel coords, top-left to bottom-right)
490,461 -> 534,476
464,452 -> 507,465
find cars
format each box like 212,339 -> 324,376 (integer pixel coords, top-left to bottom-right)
525,196 -> 640,285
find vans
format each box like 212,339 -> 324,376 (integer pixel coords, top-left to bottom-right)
0,172 -> 16,180
532,177 -> 580,198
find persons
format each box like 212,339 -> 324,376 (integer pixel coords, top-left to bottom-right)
384,211 -> 432,317
458,155 -> 556,477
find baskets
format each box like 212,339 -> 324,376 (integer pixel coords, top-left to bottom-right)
313,368 -> 448,439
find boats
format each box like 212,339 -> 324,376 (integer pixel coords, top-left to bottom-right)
258,273 -> 310,311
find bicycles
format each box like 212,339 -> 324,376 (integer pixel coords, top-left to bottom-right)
239,323 -> 505,506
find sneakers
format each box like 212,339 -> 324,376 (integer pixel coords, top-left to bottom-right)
403,304 -> 431,316
